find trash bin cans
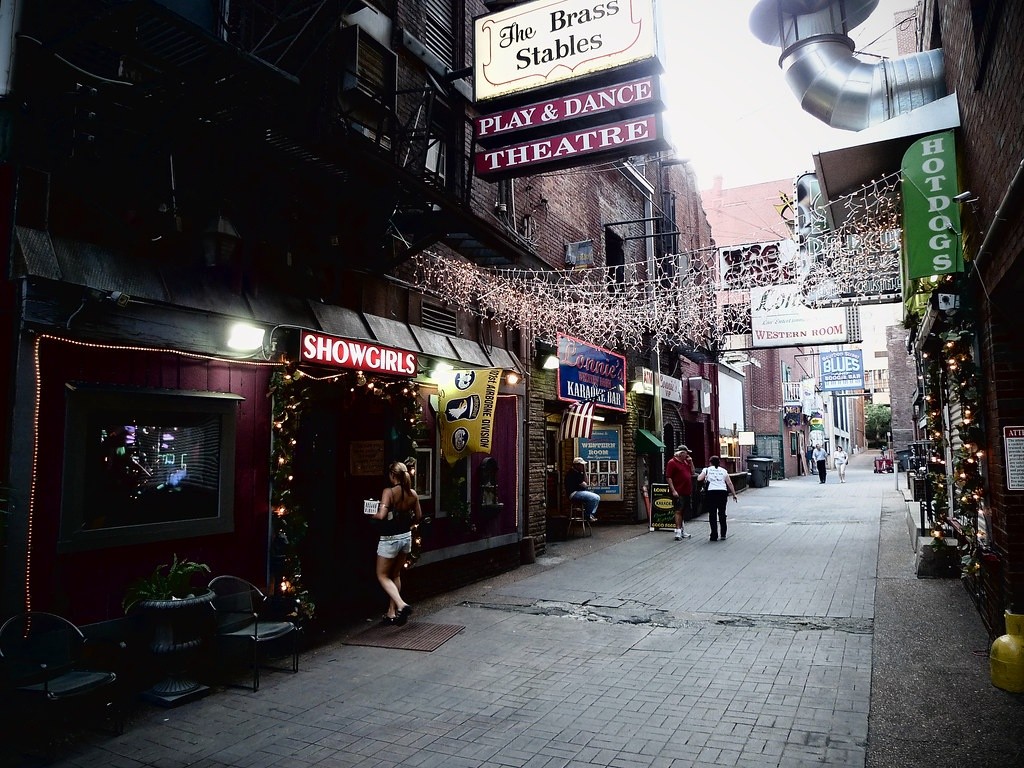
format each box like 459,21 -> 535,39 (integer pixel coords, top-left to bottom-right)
895,449 -> 914,472
690,472 -> 705,517
747,458 -> 774,488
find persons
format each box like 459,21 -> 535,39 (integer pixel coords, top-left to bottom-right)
697,454 -> 738,541
813,443 -> 848,484
566,456 -> 602,522
667,444 -> 695,541
374,461 -> 424,626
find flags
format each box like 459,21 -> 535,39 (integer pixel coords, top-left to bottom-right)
556,387 -> 613,443
438,367 -> 503,465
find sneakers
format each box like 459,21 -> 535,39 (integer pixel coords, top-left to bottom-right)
675,532 -> 682,541
682,531 -> 691,538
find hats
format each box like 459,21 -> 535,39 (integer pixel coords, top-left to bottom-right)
573,457 -> 587,465
678,445 -> 692,452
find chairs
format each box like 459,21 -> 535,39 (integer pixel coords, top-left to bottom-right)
208,574 -> 302,692
0,611 -> 124,754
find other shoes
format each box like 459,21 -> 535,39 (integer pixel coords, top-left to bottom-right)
710,536 -> 717,541
820,481 -> 825,484
588,514 -> 597,522
721,536 -> 726,540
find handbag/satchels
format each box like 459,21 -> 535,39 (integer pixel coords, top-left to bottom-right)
698,470 -> 708,495
845,456 -> 848,464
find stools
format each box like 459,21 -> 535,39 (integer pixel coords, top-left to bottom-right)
568,503 -> 593,537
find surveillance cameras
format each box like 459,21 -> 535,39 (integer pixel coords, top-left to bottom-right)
111,291 -> 129,307
952,191 -> 972,203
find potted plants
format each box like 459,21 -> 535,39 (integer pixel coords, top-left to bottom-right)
120,552 -> 211,701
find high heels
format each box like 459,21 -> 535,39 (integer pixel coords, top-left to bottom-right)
394,606 -> 413,627
382,614 -> 396,626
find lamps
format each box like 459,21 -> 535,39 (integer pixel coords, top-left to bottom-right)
203,209 -> 240,270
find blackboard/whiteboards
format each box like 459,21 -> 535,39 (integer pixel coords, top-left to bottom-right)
648,484 -> 685,529
